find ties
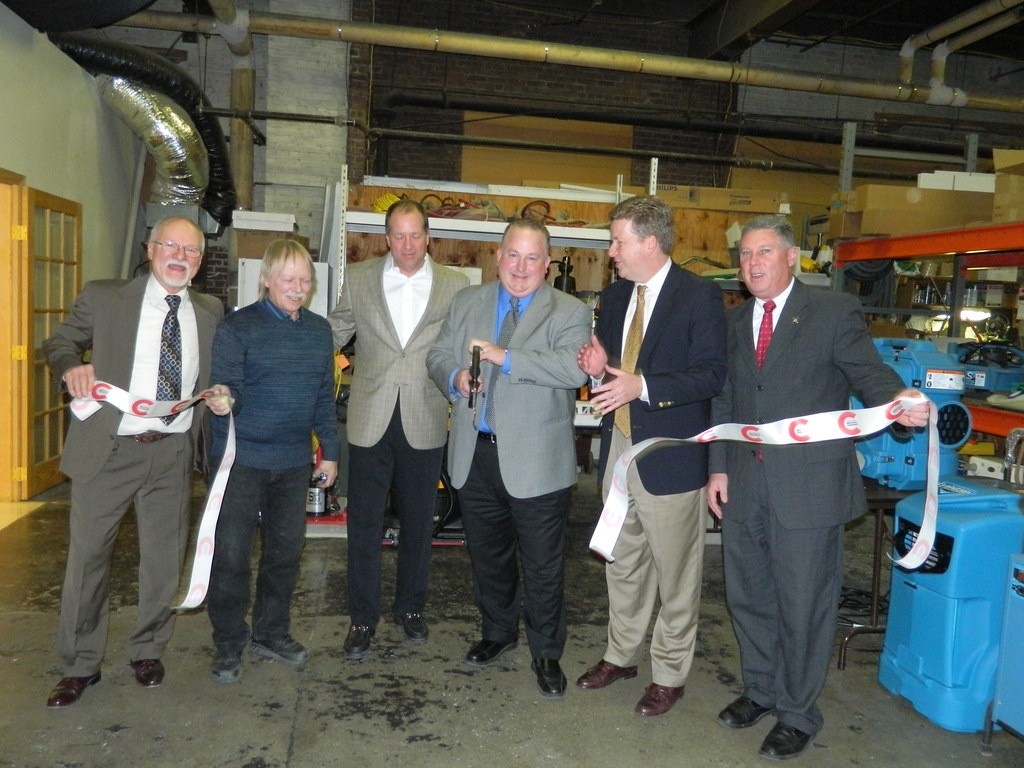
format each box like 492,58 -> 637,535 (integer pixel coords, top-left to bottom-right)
615,283 -> 646,438
155,297 -> 182,427
756,300 -> 775,369
483,296 -> 520,437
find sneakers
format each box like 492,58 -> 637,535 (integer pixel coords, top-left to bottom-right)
256,636 -> 308,665
211,645 -> 244,684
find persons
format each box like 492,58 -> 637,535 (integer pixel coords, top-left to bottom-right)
205,238 -> 340,684
705,215 -> 930,759
42,214 -> 223,710
576,194 -> 728,717
325,199 -> 471,660
425,216 -> 593,697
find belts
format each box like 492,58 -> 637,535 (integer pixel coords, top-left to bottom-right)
476,430 -> 499,444
131,433 -> 177,443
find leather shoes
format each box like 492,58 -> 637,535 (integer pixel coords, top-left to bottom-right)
635,677 -> 685,716
532,653 -> 565,699
48,672 -> 102,708
717,691 -> 775,730
758,711 -> 816,761
343,622 -> 376,660
574,654 -> 636,690
466,637 -> 519,667
130,655 -> 165,686
395,610 -> 428,643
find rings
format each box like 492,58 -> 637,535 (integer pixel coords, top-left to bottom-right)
612,397 -> 616,403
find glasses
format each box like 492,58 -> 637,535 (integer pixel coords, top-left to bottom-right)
151,240 -> 205,258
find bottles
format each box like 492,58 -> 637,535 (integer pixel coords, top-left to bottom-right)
307,453 -> 328,518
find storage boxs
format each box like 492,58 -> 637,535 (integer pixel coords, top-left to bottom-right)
825,148 -> 1024,239
647,183 -> 784,213
